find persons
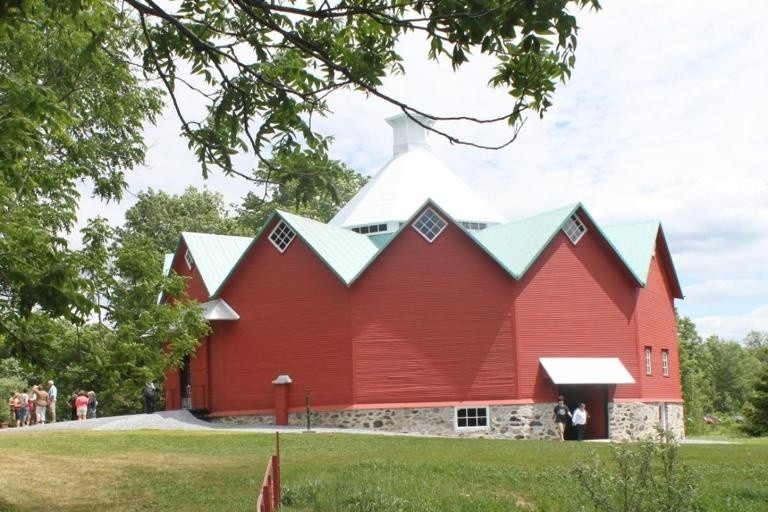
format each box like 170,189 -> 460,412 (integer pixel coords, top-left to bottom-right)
65,389 -> 99,420
552,395 -> 572,441
9,379 -> 57,428
144,380 -> 156,413
572,402 -> 590,441
